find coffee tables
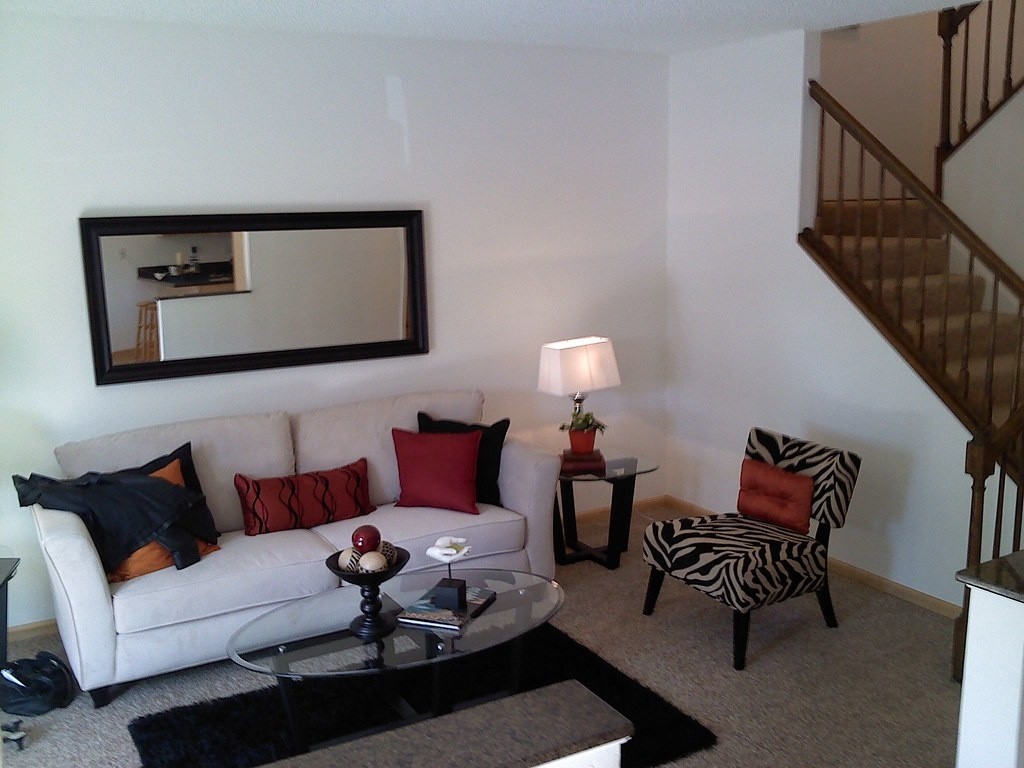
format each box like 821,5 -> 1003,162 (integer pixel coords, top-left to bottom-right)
225,568 -> 566,699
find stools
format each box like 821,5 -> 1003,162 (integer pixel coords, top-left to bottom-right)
135,301 -> 159,363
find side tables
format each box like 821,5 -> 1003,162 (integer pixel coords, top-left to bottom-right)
553,454 -> 660,570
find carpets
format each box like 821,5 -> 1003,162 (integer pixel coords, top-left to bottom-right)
127,622 -> 719,768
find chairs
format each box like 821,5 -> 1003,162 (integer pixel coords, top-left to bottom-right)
642,428 -> 863,670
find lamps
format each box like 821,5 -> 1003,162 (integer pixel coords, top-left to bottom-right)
558,410 -> 607,453
537,336 -> 621,414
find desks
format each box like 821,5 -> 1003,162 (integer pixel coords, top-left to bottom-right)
137,261 -> 235,299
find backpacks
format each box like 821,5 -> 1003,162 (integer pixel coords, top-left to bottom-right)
0,651 -> 77,716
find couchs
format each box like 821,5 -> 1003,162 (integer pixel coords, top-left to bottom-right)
30,386 -> 562,709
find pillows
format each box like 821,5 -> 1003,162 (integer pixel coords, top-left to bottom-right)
233,457 -> 377,537
88,438 -> 221,582
417,409 -> 511,509
391,427 -> 483,515
738,460 -> 812,534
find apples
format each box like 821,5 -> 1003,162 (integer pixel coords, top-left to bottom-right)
351,524 -> 380,553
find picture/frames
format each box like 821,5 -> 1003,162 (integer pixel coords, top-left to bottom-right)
76,210 -> 430,385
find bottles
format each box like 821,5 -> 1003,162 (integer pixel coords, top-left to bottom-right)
189,246 -> 200,273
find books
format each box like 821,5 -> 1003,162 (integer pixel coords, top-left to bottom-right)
396,586 -> 496,630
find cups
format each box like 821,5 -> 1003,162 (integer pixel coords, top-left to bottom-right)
168,266 -> 181,276
